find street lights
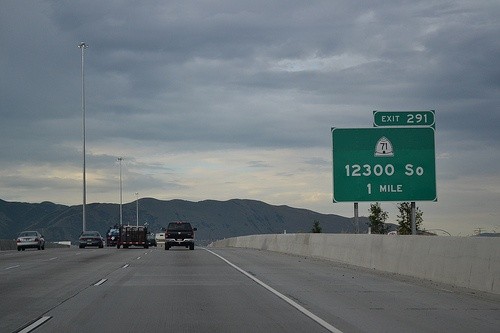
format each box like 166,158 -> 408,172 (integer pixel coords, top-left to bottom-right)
77,41 -> 89,232
135,192 -> 139,227
118,157 -> 123,226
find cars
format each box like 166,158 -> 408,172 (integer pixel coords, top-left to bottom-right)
104,225 -> 157,250
16,230 -> 46,251
78,231 -> 104,249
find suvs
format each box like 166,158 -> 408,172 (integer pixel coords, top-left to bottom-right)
161,220 -> 198,250
